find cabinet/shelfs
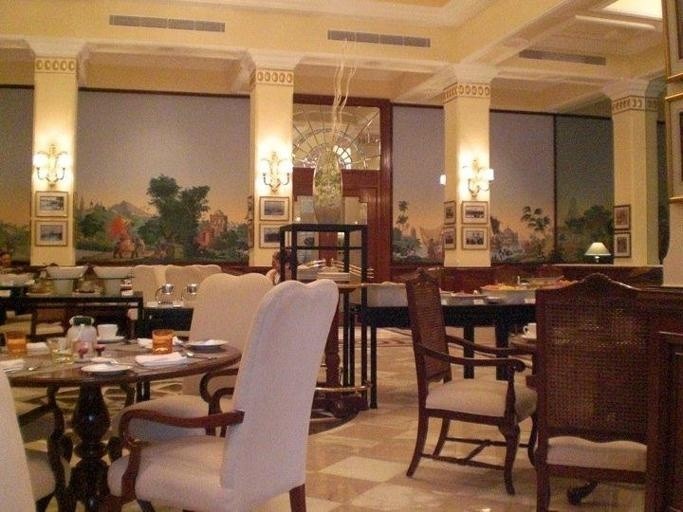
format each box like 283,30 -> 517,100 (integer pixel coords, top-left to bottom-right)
280,224 -> 369,411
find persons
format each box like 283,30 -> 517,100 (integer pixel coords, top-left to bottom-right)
0,251 -> 13,266
271,253 -> 317,285
113,237 -> 124,258
129,239 -> 140,259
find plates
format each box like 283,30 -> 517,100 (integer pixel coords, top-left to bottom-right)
520,334 -> 537,341
190,339 -> 227,352
95,337 -> 126,343
80,364 -> 133,376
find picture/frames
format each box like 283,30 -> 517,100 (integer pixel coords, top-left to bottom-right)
35,190 -> 69,218
612,204 -> 632,258
660,0 -> 683,203
258,196 -> 290,221
35,219 -> 68,246
443,199 -> 488,251
258,224 -> 292,249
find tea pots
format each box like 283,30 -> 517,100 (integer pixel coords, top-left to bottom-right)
155,284 -> 175,305
180,284 -> 197,306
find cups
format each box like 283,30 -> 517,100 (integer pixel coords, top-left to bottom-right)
522,323 -> 537,335
151,328 -> 175,352
96,324 -> 118,337
3,328 -> 28,358
46,337 -> 75,365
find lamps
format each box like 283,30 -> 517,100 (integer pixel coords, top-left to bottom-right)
460,158 -> 495,197
584,242 -> 612,263
258,151 -> 292,193
33,145 -> 68,188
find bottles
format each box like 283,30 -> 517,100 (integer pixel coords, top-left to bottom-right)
72,323 -> 95,363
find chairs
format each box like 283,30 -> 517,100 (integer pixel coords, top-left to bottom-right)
0,263 -> 339,509
321,262 -> 681,511
